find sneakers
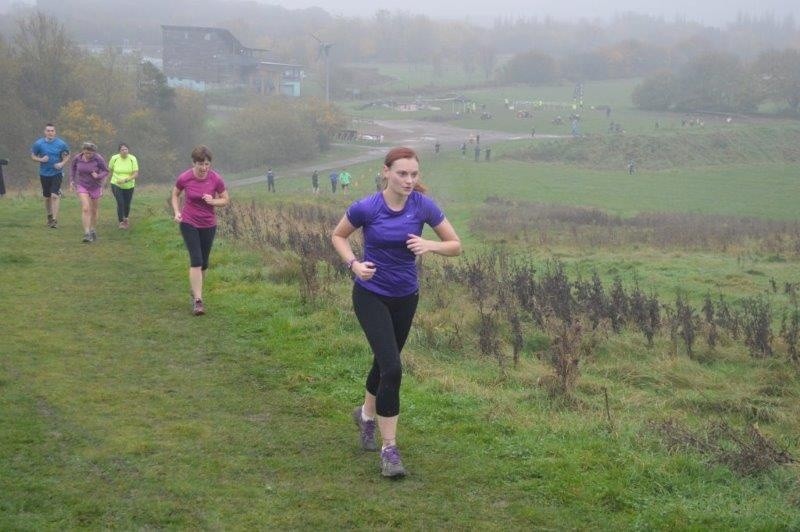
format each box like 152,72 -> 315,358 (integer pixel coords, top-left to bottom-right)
118,218 -> 129,230
352,406 -> 378,451
193,296 -> 204,316
82,229 -> 97,242
380,444 -> 405,477
47,215 -> 58,228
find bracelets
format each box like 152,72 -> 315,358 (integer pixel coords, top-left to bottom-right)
124,178 -> 128,182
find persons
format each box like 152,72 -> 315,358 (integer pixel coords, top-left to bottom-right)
330,147 -> 463,479
172,146 -> 229,317
375,171 -> 382,192
330,170 -> 338,193
106,143 -> 139,230
29,123 -> 71,228
339,168 -> 352,194
266,168 -> 276,193
69,142 -> 109,243
434,94 -> 733,175
312,170 -> 319,191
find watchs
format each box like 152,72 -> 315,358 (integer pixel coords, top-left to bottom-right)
346,259 -> 357,270
